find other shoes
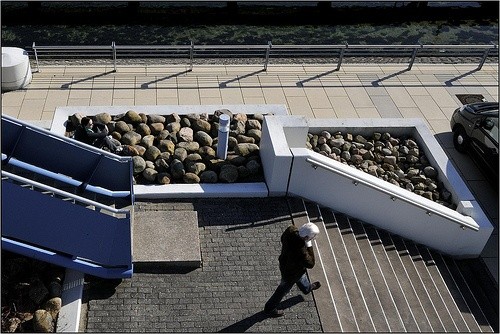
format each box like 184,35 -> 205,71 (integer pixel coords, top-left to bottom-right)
305,281 -> 321,295
266,307 -> 284,316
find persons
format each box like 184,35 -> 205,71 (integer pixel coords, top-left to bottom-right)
74,116 -> 120,153
264,221 -> 322,316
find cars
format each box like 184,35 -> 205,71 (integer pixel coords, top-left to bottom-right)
450,101 -> 499,193
